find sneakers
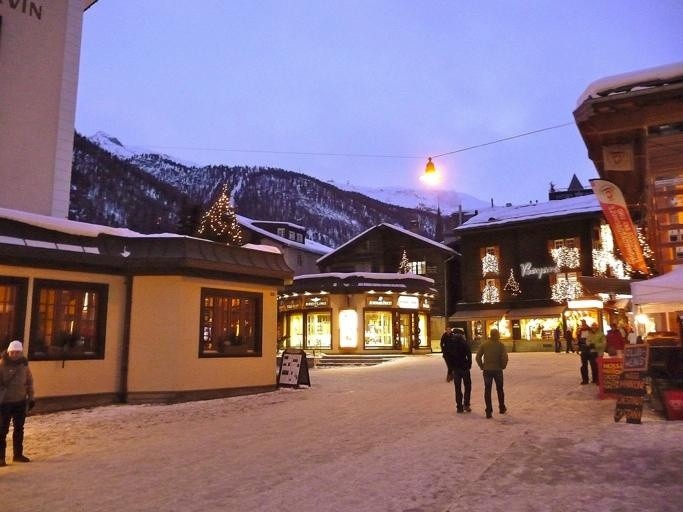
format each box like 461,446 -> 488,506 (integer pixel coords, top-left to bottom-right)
581,382 -> 588,384
13,455 -> 29,462
487,408 -> 506,418
457,406 -> 471,413
1,458 -> 6,466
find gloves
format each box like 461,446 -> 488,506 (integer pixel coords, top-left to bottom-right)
28,400 -> 35,411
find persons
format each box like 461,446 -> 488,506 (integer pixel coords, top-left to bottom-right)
605,322 -> 624,356
553,325 -> 562,353
578,319 -> 590,385
474,328 -> 507,419
563,326 -> 576,353
584,320 -> 606,386
443,328 -> 472,414
0,339 -> 35,468
440,326 -> 454,383
575,323 -> 582,346
622,324 -> 644,344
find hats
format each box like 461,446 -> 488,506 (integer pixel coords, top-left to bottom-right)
453,329 -> 464,335
7,340 -> 23,353
491,329 -> 500,338
